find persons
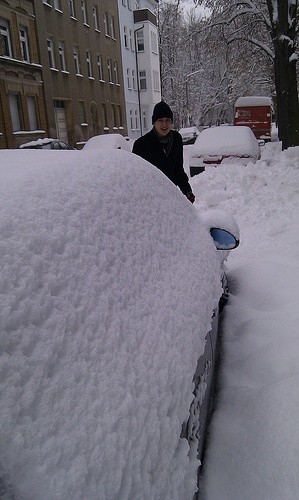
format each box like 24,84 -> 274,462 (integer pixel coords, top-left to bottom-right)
132,101 -> 195,204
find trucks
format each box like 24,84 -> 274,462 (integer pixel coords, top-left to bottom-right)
233,97 -> 273,143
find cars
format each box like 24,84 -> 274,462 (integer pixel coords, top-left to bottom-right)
179,127 -> 199,146
189,126 -> 266,176
18,139 -> 76,150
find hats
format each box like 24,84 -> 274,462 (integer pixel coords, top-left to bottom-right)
152,101 -> 173,125
